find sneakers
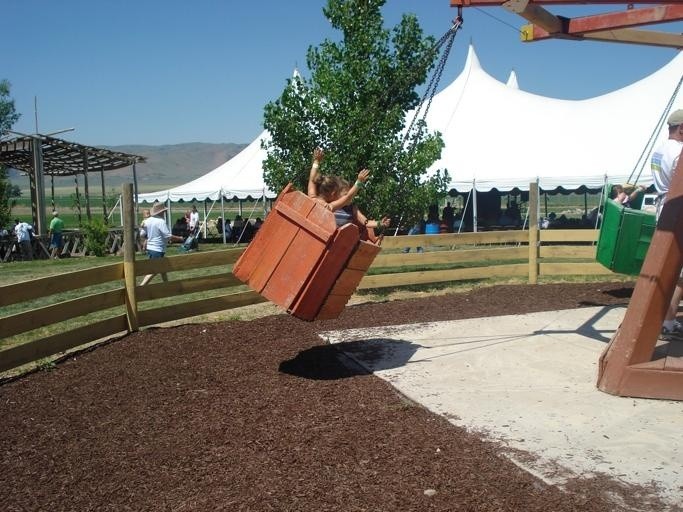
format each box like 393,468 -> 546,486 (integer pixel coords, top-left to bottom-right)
658,322 -> 683,342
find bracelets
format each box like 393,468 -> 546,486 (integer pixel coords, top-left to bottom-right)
354,181 -> 362,188
635,189 -> 640,193
312,164 -> 319,168
376,221 -> 382,229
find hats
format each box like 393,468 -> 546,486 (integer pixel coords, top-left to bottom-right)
667,108 -> 683,127
149,202 -> 169,217
51,210 -> 58,216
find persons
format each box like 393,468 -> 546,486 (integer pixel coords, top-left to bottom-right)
135,209 -> 151,256
140,202 -> 185,285
610,184 -> 645,210
333,179 -> 391,228
47,211 -> 66,260
185,209 -> 192,224
215,215 -> 265,244
308,146 -> 370,210
188,205 -> 199,238
11,218 -> 34,260
650,110 -> 683,342
443,201 -> 457,223
23,221 -> 41,260
